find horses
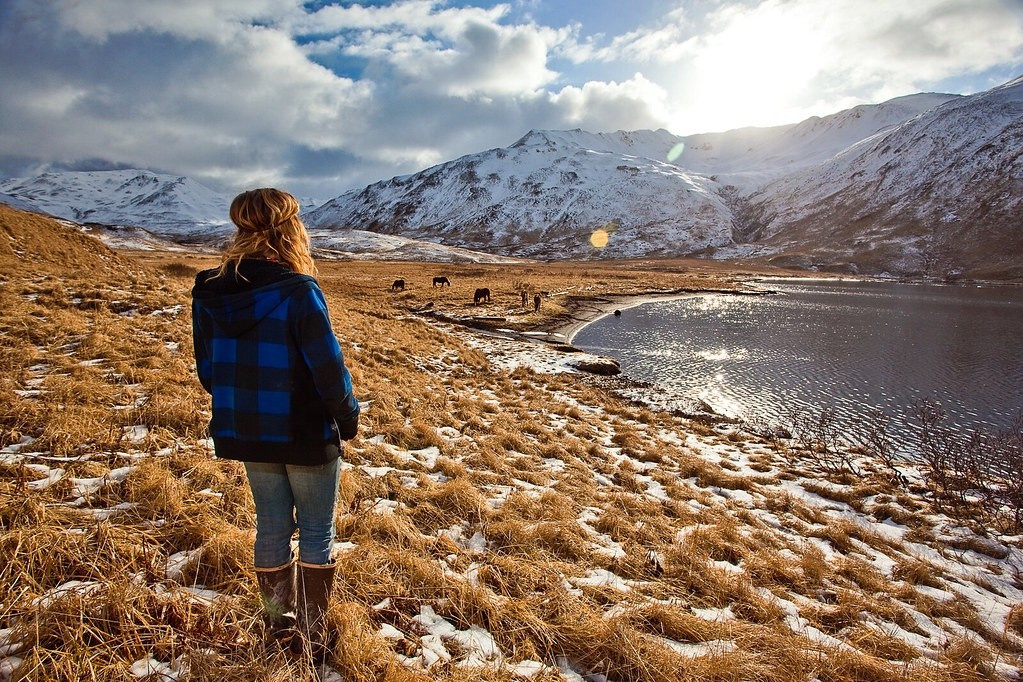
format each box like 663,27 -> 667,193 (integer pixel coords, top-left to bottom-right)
474,287 -> 490,305
432,277 -> 450,287
392,280 -> 404,290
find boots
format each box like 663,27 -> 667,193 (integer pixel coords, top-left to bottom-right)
289,559 -> 334,663
254,552 -> 298,644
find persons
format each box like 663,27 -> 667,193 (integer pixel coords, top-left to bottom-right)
191,189 -> 359,654
534,292 -> 545,310
521,289 -> 530,306
